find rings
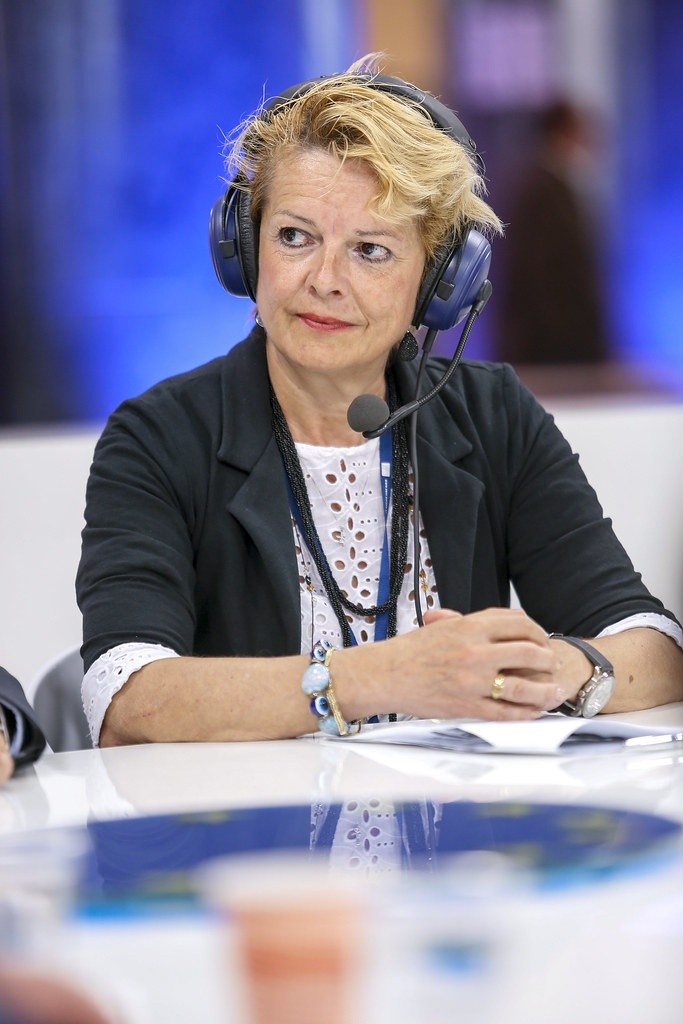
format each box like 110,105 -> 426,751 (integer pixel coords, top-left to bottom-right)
491,673 -> 505,699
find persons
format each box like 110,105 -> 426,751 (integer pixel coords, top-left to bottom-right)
496,91 -> 609,371
74,52 -> 683,750
0,666 -> 47,786
0,968 -> 111,1024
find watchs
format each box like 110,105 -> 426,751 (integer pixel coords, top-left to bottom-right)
546,632 -> 615,718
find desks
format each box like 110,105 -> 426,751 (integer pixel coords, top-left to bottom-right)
0,739 -> 683,1024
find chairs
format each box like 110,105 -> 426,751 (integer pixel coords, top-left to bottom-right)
32,648 -> 92,754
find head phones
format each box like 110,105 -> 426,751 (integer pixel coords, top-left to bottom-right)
209,70 -> 493,333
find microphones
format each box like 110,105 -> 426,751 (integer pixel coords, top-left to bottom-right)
346,312 -> 478,440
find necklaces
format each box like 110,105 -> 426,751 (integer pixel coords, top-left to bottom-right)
268,360 -> 412,722
298,436 -> 378,544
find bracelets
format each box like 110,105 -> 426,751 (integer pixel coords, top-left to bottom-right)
302,639 -> 362,736
